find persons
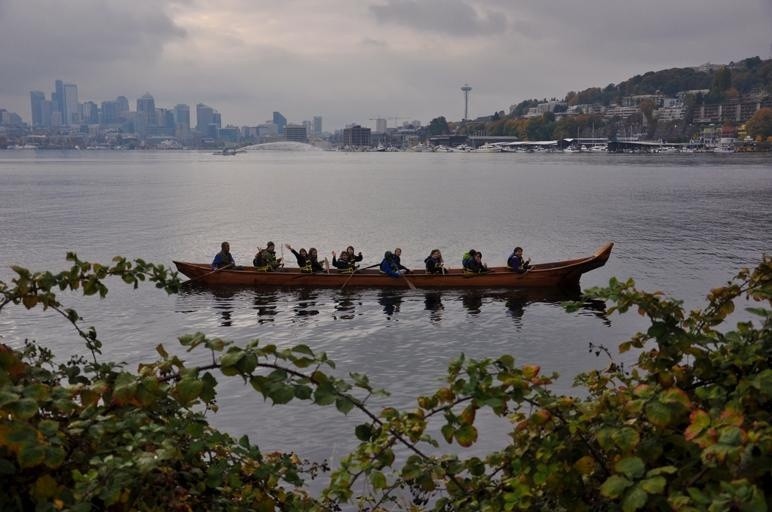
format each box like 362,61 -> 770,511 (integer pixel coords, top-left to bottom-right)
284,243 -> 329,273
424,248 -> 450,275
378,247 -> 409,275
331,249 -> 355,275
461,249 -> 496,275
253,240 -> 284,273
210,242 -> 244,273
338,245 -> 363,274
308,247 -> 325,273
505,246 -> 532,273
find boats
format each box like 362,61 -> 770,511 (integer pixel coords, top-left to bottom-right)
212,143 -> 244,156
172,242 -> 616,288
179,277 -> 614,332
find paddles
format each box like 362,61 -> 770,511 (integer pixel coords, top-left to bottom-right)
281,242 -> 284,265
357,263 -> 382,272
395,265 -> 417,291
181,261 -> 235,287
324,256 -> 330,274
515,264 -> 535,280
338,264 -> 360,290
